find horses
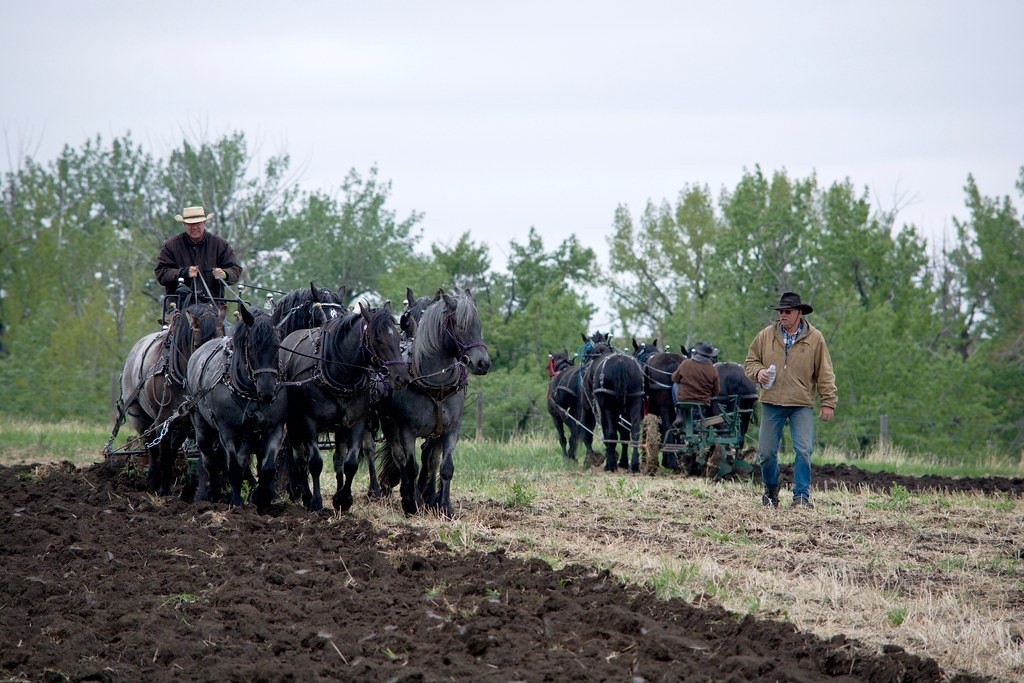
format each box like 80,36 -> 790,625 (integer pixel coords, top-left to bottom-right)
545,329 -> 758,475
118,280 -> 493,520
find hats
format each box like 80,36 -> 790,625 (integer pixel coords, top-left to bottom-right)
768,292 -> 814,315
695,342 -> 720,356
173,207 -> 215,224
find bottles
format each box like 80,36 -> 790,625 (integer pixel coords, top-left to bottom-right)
762,365 -> 776,389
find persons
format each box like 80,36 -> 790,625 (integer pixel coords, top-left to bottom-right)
669,342 -> 721,422
743,292 -> 839,509
155,205 -> 243,311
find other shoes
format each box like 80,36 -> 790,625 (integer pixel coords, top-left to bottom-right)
791,498 -> 814,509
762,485 -> 780,508
672,416 -> 683,427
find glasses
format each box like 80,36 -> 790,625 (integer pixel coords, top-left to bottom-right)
780,308 -> 798,315
